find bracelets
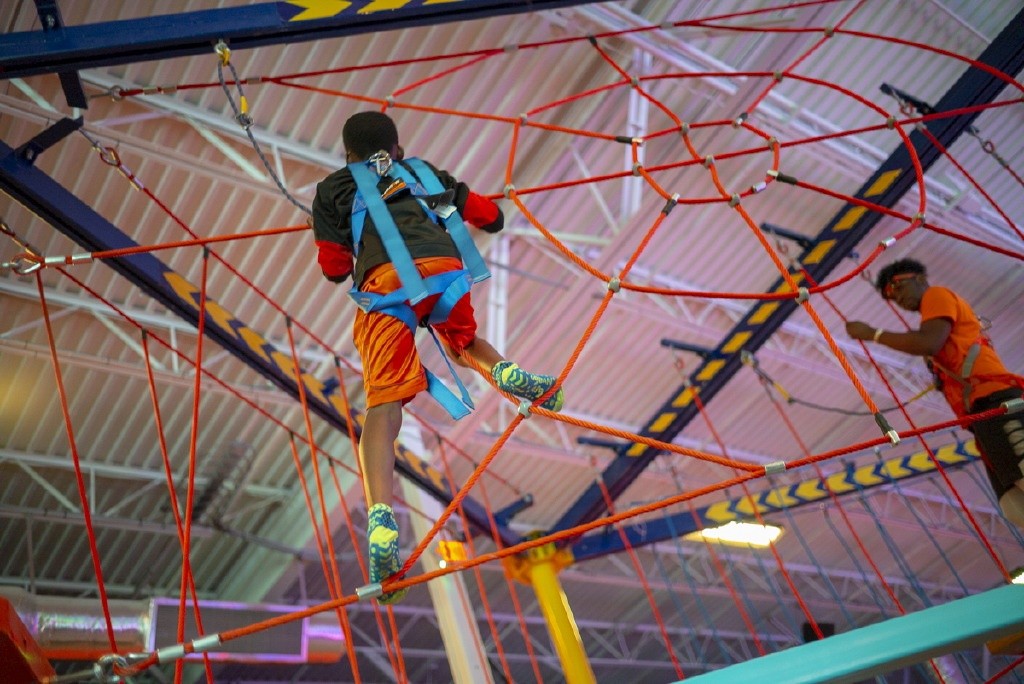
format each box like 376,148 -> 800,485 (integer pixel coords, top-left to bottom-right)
874,328 -> 885,343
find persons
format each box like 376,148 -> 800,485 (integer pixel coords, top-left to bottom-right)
845,257 -> 1024,540
312,110 -> 566,605
